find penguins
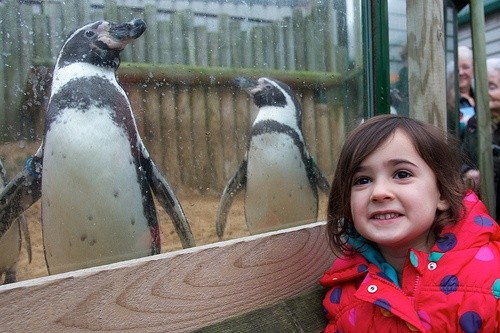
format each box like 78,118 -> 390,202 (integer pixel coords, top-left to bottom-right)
215,77 -> 332,240
1,18 -> 198,278
0,160 -> 33,285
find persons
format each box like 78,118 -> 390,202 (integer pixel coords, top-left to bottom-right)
316,114 -> 500,333
387,50 -> 410,118
447,46 -> 477,139
459,57 -> 500,224
